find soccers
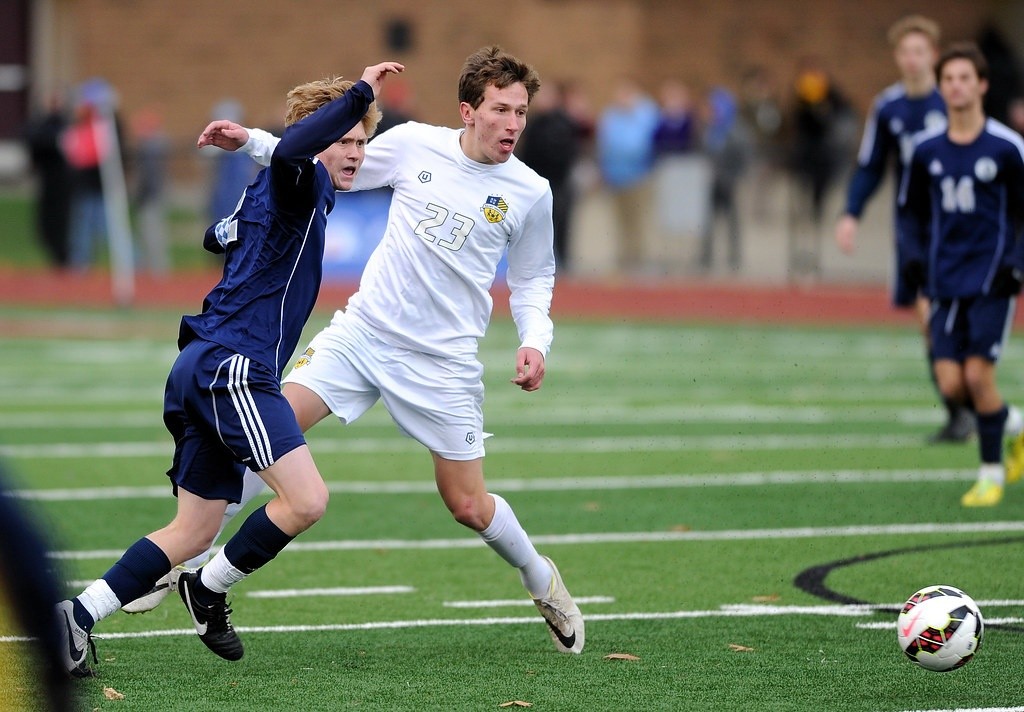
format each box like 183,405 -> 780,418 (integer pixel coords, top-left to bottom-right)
897,584 -> 984,671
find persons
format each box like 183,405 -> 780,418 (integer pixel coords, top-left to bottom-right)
892,44 -> 1024,509
835,14 -> 978,447
0,467 -> 88,712
53,60 -> 405,684
16,54 -> 866,281
936,23 -> 1023,137
123,48 -> 586,655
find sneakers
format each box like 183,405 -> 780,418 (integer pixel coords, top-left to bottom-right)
119,560 -> 209,614
1003,417 -> 1024,479
177,570 -> 243,661
526,553 -> 583,654
934,413 -> 974,444
54,598 -> 102,680
957,462 -> 1003,508
